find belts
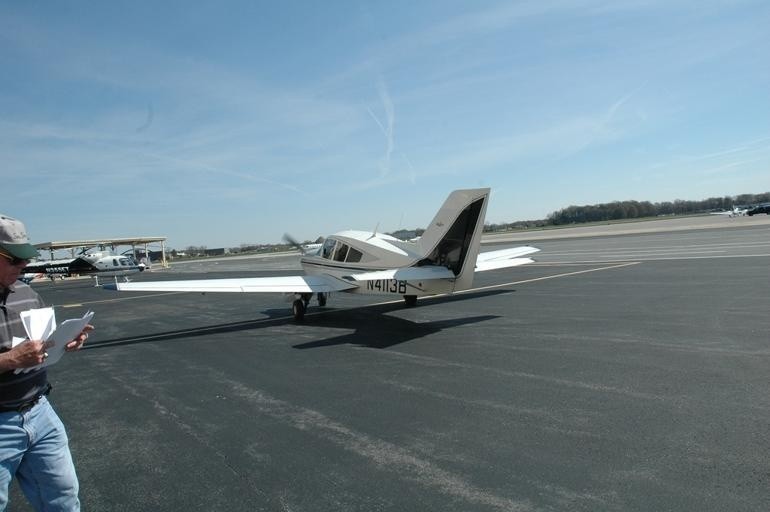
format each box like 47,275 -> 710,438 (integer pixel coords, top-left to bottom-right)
0,402 -> 32,412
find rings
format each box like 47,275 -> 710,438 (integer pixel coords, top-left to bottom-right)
42,352 -> 49,360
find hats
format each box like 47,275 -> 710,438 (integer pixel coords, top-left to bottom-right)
0,215 -> 41,259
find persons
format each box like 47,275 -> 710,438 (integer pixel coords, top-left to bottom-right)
1,215 -> 96,512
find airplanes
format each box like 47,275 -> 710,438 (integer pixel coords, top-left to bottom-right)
100,187 -> 541,320
20,249 -> 146,289
709,205 -> 750,218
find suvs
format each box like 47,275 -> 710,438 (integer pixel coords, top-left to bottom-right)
747,205 -> 770,216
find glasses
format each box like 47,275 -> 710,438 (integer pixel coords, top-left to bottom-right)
0,253 -> 22,265
